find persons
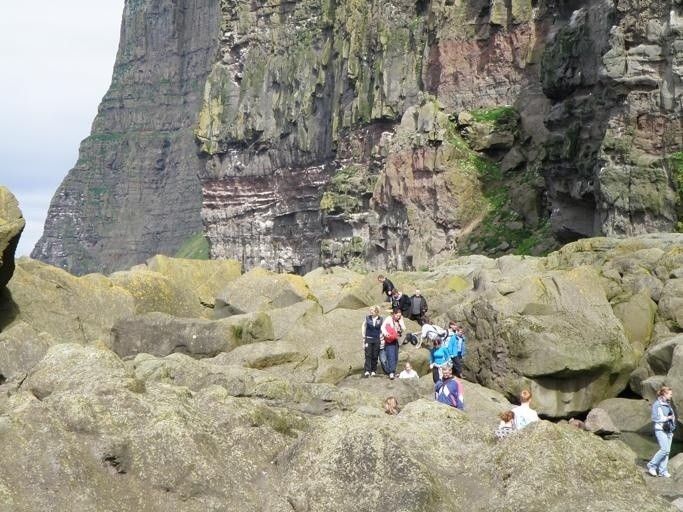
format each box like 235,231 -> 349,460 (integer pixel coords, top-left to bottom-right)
381,397 -> 404,418
494,410 -> 519,444
647,386 -> 677,479
433,365 -> 466,412
509,389 -> 540,430
360,273 -> 467,384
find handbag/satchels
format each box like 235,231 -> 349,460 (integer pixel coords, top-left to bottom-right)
662,412 -> 677,433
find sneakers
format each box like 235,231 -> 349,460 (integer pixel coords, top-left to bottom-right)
364,372 -> 369,377
389,373 -> 394,380
658,472 -> 670,478
648,469 -> 656,477
370,372 -> 375,377
